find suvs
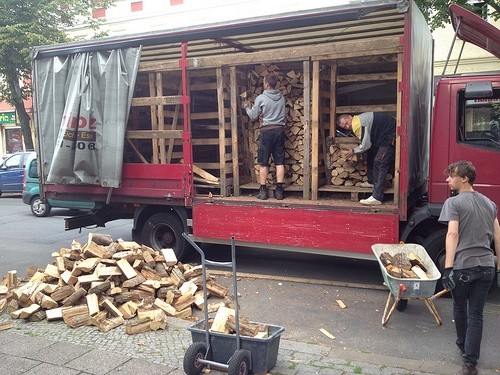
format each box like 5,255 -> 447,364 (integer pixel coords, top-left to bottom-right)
0,149 -> 35,197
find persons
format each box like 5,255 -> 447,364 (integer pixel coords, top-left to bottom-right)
241,73 -> 286,200
439,160 -> 500,375
336,111 -> 396,205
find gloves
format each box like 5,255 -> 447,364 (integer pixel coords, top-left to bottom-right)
442,267 -> 456,290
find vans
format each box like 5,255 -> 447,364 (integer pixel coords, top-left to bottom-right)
22,155 -> 95,217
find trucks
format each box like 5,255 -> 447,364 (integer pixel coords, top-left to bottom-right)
24,0 -> 500,297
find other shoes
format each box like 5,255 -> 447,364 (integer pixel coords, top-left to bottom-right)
256,190 -> 269,200
457,346 -> 465,354
275,189 -> 284,199
462,362 -> 477,375
360,196 -> 382,204
360,181 -> 374,187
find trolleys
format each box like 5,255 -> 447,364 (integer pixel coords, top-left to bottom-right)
371,244 -> 451,327
182,233 -> 252,375
